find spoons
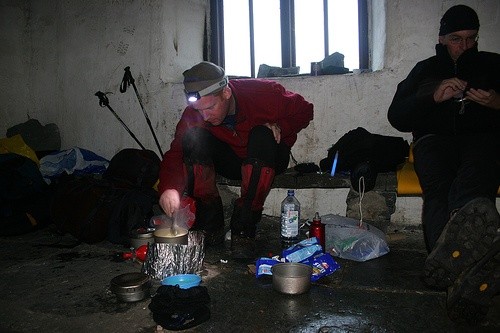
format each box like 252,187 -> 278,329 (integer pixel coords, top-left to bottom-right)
169,209 -> 180,236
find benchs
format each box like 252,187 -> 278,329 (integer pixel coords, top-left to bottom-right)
214,166 -> 423,230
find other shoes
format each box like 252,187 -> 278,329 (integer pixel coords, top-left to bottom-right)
231,230 -> 256,261
204,230 -> 225,263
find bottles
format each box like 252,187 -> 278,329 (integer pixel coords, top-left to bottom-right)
281,189 -> 301,242
309,212 -> 325,253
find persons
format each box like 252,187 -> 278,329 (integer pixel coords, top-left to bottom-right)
157,62 -> 315,262
387,5 -> 500,327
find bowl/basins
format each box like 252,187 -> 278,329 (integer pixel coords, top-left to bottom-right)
124,226 -> 156,248
160,274 -> 202,288
108,272 -> 153,304
153,227 -> 190,245
270,262 -> 313,294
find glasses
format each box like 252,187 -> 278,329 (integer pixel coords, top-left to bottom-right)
445,34 -> 479,43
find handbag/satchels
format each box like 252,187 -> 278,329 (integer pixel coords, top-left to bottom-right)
0,134 -> 40,169
102,149 -> 161,189
39,146 -> 110,185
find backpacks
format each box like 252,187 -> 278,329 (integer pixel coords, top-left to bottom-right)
0,153 -> 51,236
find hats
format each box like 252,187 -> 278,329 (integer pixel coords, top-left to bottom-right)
439,5 -> 480,36
183,61 -> 228,96
